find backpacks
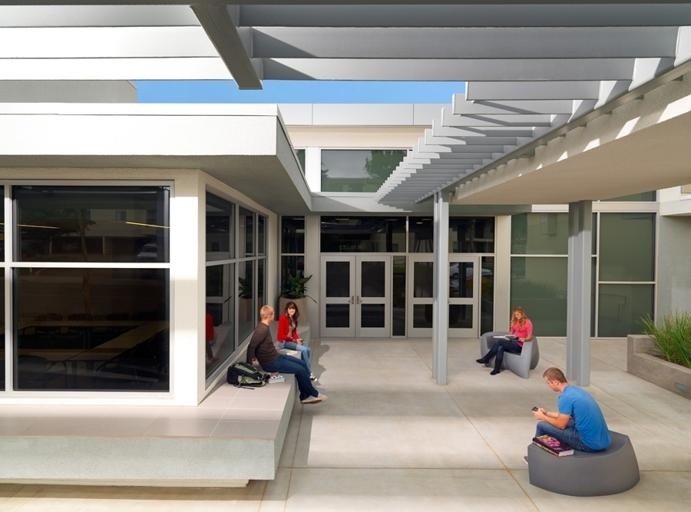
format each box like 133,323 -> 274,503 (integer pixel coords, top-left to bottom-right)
227,361 -> 270,390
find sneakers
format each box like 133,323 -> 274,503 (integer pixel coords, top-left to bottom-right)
300,393 -> 329,404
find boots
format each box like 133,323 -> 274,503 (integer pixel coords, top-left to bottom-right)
476,350 -> 503,375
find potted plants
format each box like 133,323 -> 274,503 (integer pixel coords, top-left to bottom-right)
279,266 -> 318,328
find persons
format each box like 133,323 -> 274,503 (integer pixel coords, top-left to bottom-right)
247,301 -> 328,404
522,367 -> 611,465
206,311 -> 218,361
476,306 -> 534,375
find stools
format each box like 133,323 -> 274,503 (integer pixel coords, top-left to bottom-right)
526,428 -> 640,498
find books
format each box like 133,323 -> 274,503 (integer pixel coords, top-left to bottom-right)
533,434 -> 575,457
492,334 -> 520,343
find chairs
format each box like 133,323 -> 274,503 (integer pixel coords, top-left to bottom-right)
479,331 -> 540,378
